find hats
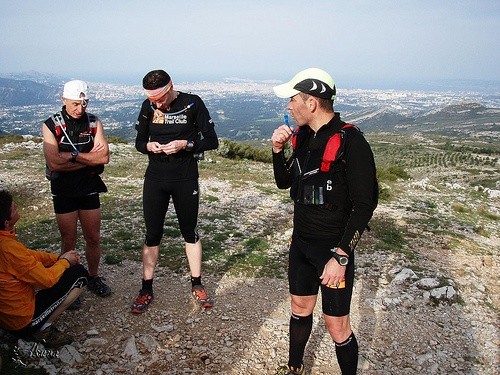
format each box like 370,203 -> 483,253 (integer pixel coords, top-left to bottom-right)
62,80 -> 89,101
274,67 -> 336,101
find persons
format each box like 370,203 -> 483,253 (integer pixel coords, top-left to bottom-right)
0,188 -> 88,350
43,80 -> 111,309
130,70 -> 214,314
270,68 -> 380,375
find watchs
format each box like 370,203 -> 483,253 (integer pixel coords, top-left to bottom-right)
71,151 -> 78,162
330,249 -> 349,267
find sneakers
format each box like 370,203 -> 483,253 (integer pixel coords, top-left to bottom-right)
31,325 -> 73,348
270,361 -> 304,375
190,284 -> 213,307
131,289 -> 154,313
87,275 -> 111,297
